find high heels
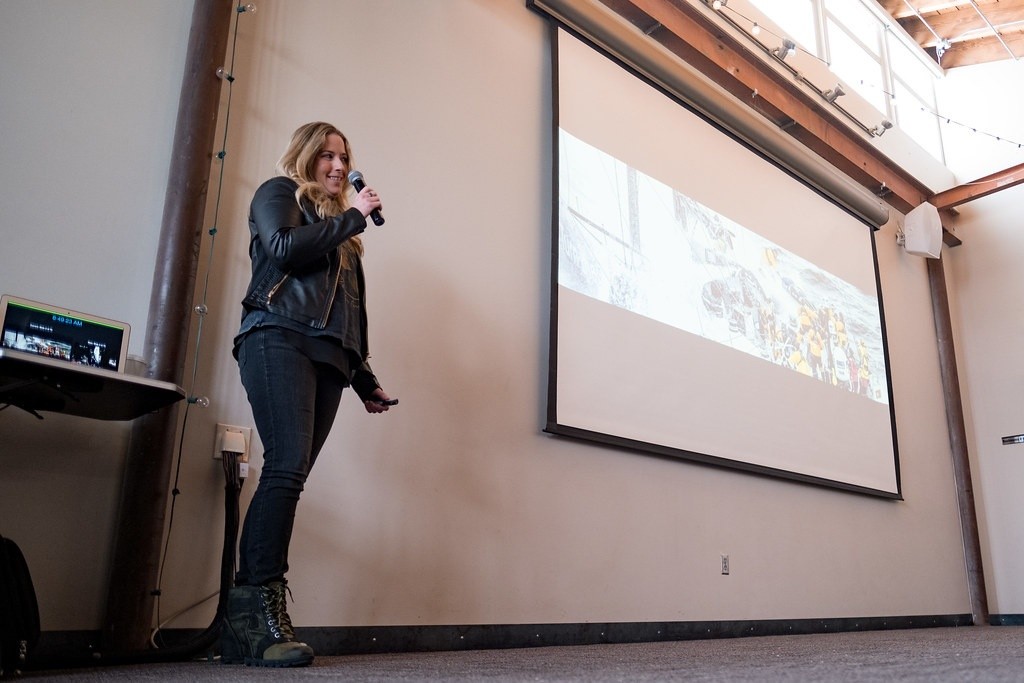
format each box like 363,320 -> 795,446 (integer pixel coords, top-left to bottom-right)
215,582 -> 313,667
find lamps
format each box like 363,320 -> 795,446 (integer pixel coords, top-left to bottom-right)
821,83 -> 847,102
869,119 -> 893,137
770,39 -> 795,59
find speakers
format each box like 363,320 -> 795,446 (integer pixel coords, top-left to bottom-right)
905,200 -> 943,260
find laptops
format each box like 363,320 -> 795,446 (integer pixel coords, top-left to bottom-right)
0,295 -> 129,372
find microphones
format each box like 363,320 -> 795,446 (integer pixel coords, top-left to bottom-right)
347,170 -> 385,226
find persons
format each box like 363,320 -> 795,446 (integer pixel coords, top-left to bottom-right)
219,120 -> 391,668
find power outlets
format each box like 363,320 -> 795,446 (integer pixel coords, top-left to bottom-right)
213,423 -> 251,462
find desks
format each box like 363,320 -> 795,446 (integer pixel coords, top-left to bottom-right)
0,347 -> 188,422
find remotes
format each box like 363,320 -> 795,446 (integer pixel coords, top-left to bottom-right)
374,399 -> 398,406
